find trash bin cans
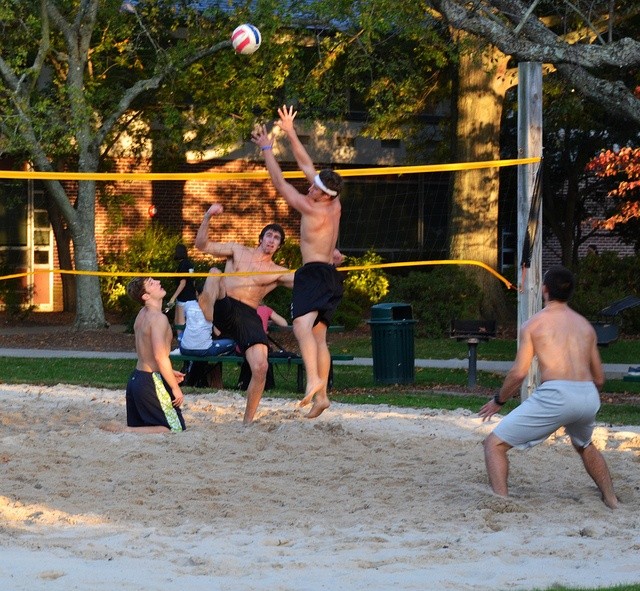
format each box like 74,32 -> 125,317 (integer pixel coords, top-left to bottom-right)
367,303 -> 418,385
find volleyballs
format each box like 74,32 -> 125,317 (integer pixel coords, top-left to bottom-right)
231,24 -> 262,54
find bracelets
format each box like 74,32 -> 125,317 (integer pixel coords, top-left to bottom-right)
203,212 -> 211,219
261,145 -> 273,150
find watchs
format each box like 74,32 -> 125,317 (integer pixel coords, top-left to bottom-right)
493,391 -> 507,407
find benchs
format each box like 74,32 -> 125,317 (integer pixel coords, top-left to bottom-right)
169,348 -> 354,394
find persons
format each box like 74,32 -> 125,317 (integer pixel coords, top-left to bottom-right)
177,280 -> 237,357
195,202 -> 345,427
169,243 -> 198,337
250,103 -> 345,419
476,263 -> 620,510
125,275 -> 187,436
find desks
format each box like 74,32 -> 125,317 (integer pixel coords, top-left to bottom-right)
267,325 -> 343,333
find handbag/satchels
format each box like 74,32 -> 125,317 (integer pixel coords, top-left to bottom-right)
268,351 -> 297,358
